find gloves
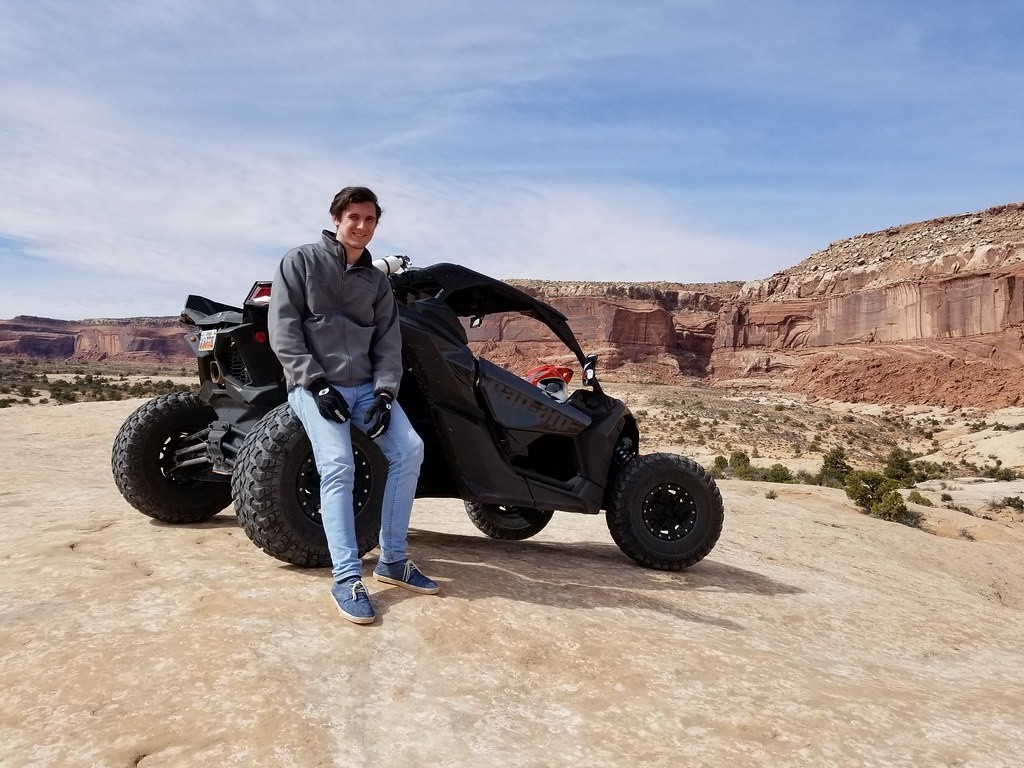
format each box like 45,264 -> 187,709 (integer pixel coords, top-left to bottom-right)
307,377 -> 352,424
364,389 -> 394,441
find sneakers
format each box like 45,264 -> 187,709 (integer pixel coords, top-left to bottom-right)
330,577 -> 376,624
372,558 -> 440,594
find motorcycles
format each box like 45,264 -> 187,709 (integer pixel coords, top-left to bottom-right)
108,255 -> 726,571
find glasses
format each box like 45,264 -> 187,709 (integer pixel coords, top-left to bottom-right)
545,383 -> 562,393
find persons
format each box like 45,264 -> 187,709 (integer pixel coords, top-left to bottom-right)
268,187 -> 441,624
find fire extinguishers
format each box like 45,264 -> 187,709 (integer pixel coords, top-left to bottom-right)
372,254 -> 412,275
